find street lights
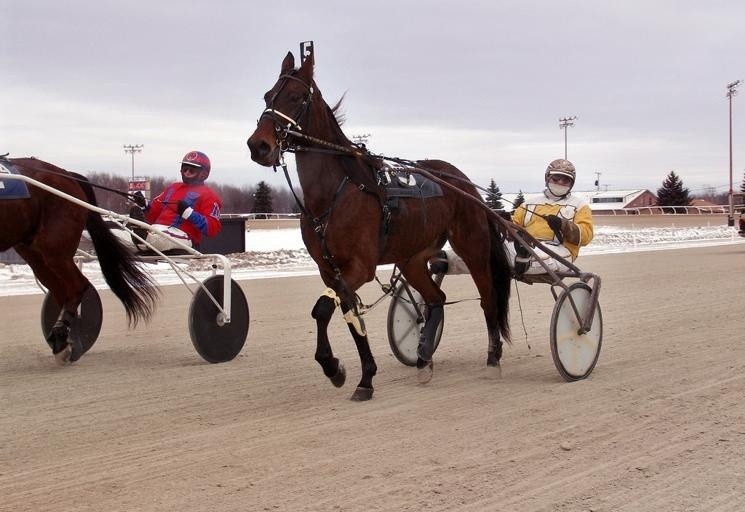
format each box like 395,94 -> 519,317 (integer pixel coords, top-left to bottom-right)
122,145 -> 146,178
724,80 -> 742,226
555,111 -> 578,161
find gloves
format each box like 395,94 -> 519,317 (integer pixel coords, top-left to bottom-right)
543,214 -> 562,230
133,191 -> 147,209
176,200 -> 189,216
500,211 -> 513,222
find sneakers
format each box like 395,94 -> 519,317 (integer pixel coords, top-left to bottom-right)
129,206 -> 149,244
428,248 -> 449,275
513,230 -> 536,274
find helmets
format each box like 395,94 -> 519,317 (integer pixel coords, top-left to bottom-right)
544,157 -> 577,197
179,150 -> 211,179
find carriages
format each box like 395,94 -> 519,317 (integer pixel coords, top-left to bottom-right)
0,152 -> 250,363
247,52 -> 604,405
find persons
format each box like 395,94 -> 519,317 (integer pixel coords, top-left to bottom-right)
74,149 -> 224,254
427,156 -> 596,279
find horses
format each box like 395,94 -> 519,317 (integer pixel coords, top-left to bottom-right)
0,157 -> 162,367
247,50 -> 512,402
